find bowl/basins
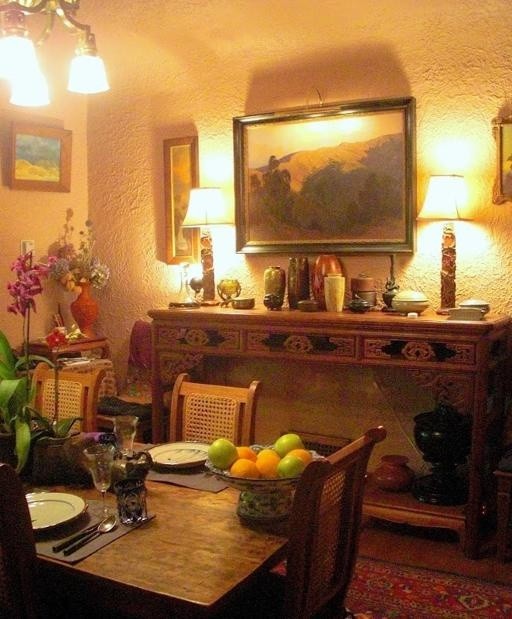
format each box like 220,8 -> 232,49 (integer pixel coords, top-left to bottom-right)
447,308 -> 482,320
232,298 -> 254,309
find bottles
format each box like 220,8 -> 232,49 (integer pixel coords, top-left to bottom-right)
323,272 -> 346,313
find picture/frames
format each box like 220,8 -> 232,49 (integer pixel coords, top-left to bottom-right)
233,96 -> 416,257
162,135 -> 200,266
490,114 -> 512,204
6,117 -> 74,195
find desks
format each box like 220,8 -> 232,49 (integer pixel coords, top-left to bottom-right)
147,309 -> 512,560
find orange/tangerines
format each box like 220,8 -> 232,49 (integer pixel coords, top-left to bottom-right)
206,432 -> 313,479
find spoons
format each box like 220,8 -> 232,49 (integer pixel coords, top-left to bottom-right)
63,513 -> 115,555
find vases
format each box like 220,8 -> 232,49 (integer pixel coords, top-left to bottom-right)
70,282 -> 98,339
259,250 -> 350,314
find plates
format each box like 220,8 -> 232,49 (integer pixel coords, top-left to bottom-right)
149,439 -> 216,468
26,491 -> 85,531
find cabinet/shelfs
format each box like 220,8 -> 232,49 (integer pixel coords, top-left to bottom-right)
25,337 -> 108,364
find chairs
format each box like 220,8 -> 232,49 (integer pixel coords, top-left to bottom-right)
280,426 -> 386,619
28,362 -> 106,435
0,463 -> 45,619
169,372 -> 262,447
282,433 -> 354,461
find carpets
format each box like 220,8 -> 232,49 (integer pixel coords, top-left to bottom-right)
268,556 -> 512,619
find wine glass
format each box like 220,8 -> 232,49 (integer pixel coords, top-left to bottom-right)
82,444 -> 115,517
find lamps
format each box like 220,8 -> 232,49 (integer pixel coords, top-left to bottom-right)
180,185 -> 234,302
0,0 -> 109,107
415,176 -> 476,314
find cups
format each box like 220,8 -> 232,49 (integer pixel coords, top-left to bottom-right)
56,327 -> 68,336
113,416 -> 138,455
113,478 -> 148,522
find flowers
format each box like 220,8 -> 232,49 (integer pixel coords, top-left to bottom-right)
46,210 -> 111,292
7,250 -> 60,415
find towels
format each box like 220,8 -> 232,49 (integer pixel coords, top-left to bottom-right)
142,469 -> 232,493
34,498 -> 155,565
